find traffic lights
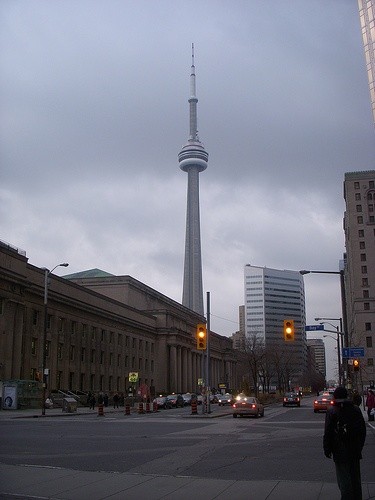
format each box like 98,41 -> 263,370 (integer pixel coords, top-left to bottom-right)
353,358 -> 359,371
284,319 -> 294,342
197,323 -> 206,350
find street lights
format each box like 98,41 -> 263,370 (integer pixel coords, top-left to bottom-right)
42,264 -> 70,416
320,321 -> 341,382
322,335 -> 341,383
298,269 -> 352,390
314,317 -> 346,390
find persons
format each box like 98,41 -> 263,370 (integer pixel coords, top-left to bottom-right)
113,393 -> 124,409
46,396 -> 53,408
366,390 -> 375,421
98,394 -> 108,407
323,386 -> 367,500
87,391 -> 96,410
348,388 -> 360,408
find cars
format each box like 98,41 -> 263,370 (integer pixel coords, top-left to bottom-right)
218,392 -> 236,406
195,394 -> 206,404
237,392 -> 246,400
319,388 -> 336,395
210,394 -> 223,403
181,392 -> 197,406
167,392 -> 185,409
233,396 -> 265,417
282,392 -> 300,407
153,395 -> 173,410
314,392 -> 334,411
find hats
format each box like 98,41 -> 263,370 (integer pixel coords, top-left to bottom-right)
333,387 -> 348,398
